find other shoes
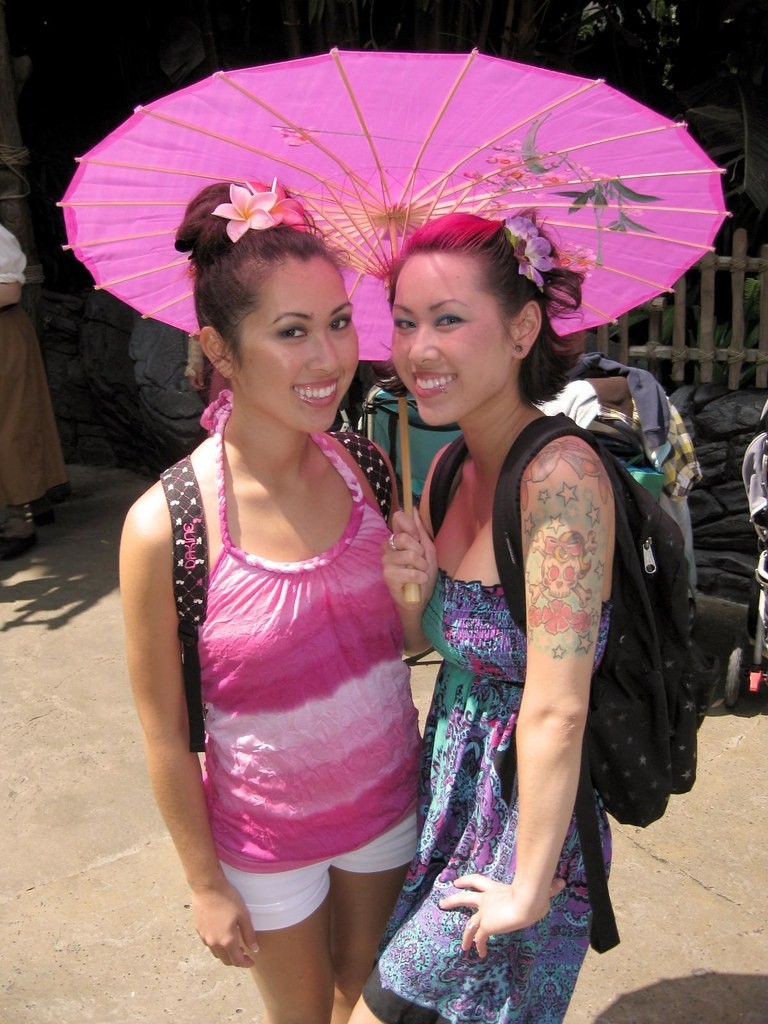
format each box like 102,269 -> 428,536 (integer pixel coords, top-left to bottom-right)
34,506 -> 55,527
0,532 -> 36,560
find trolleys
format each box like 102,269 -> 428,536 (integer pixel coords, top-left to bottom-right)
723,401 -> 767,709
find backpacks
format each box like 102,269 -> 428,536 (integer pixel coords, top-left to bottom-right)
430,423 -> 720,826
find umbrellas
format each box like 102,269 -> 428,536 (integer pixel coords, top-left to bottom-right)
68,45 -> 736,607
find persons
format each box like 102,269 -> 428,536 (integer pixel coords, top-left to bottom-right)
379,207 -> 626,1024
0,224 -> 73,562
114,168 -> 441,1024
185,333 -> 232,409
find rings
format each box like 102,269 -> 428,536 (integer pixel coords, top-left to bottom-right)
389,533 -> 397,550
